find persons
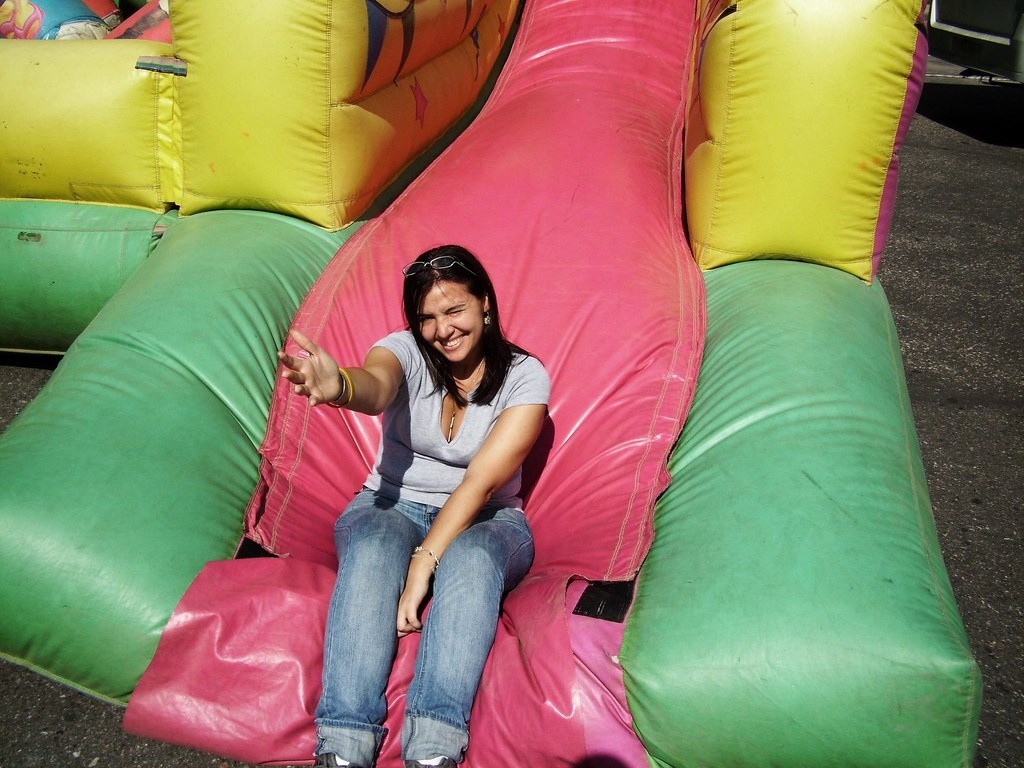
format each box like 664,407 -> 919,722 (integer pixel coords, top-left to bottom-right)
277,246 -> 551,768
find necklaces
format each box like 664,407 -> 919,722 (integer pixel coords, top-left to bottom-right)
447,356 -> 484,442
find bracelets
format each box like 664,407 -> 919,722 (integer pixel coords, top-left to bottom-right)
414,547 -> 440,565
412,554 -> 435,574
325,369 -> 353,408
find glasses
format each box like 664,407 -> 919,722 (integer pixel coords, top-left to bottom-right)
402,255 -> 477,281
